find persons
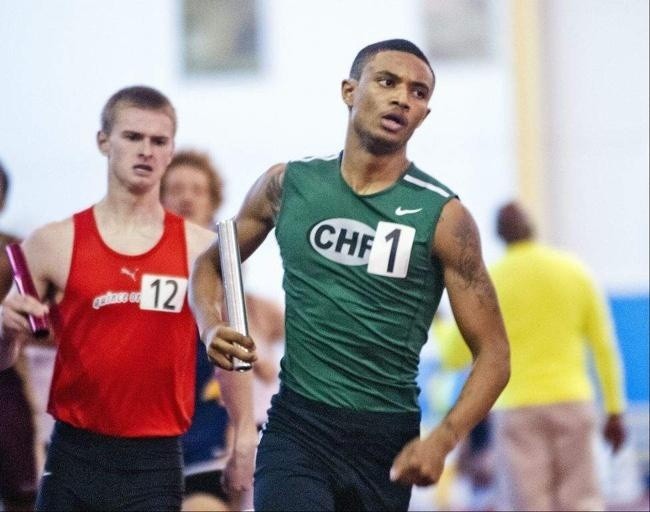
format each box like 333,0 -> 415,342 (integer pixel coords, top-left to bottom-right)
0,169 -> 41,509
159,149 -> 288,512
1,85 -> 261,512
186,38 -> 513,510
434,201 -> 628,511
